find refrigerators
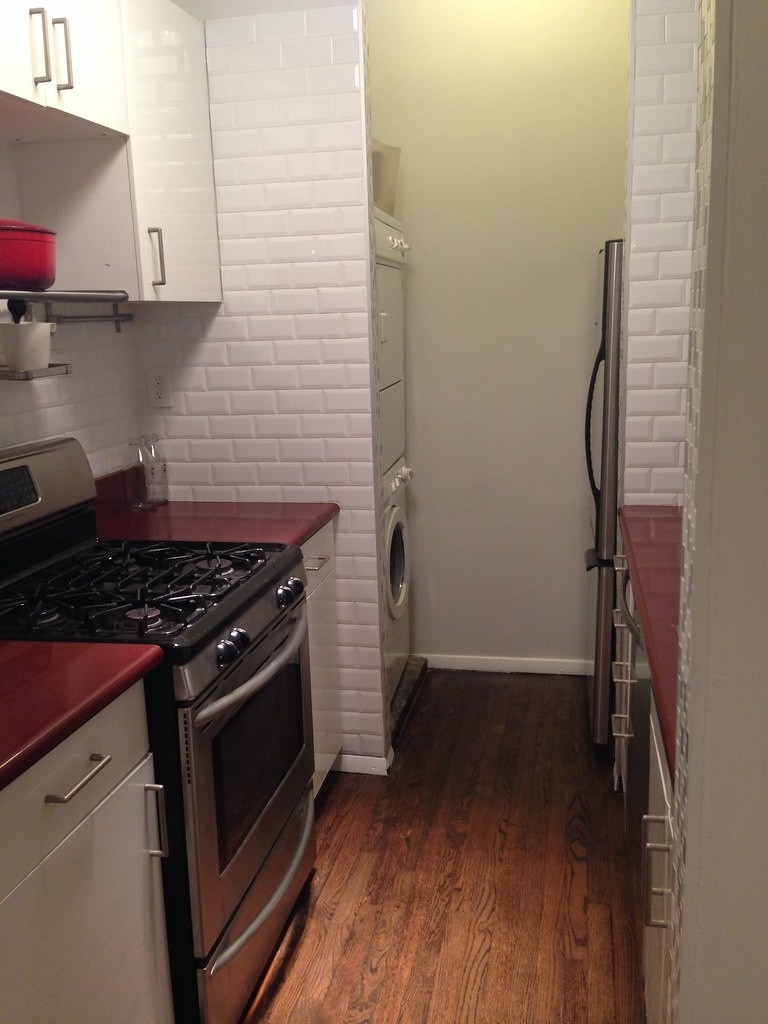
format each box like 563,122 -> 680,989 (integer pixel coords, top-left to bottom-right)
582,239 -> 617,744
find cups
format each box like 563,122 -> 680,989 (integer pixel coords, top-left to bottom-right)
0,322 -> 57,372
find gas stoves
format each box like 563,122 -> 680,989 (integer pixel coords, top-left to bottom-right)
0,435 -> 309,699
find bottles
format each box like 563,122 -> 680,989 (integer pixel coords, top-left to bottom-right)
124,437 -> 156,512
141,437 -> 168,506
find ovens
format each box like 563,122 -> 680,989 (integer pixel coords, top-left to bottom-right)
149,602 -> 316,1024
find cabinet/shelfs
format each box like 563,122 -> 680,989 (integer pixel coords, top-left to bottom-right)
1,0 -> 227,305
0,637 -> 180,1024
93,464 -> 347,799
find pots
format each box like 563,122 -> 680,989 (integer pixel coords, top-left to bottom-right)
0,217 -> 56,291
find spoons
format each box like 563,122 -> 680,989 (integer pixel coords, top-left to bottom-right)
7,298 -> 27,323
